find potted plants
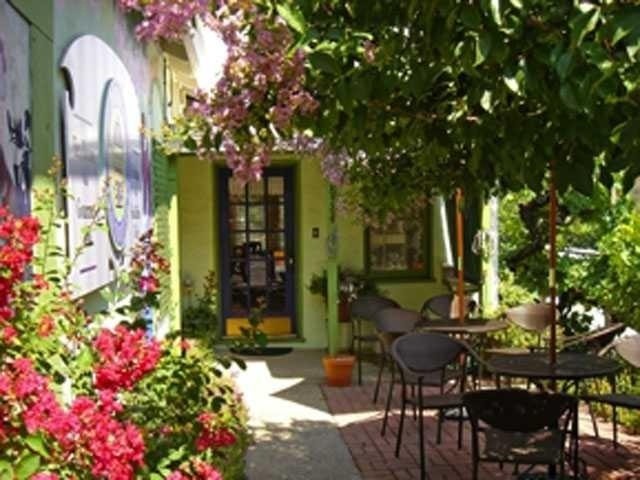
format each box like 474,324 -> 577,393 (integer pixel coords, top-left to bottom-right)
309,268 -> 377,320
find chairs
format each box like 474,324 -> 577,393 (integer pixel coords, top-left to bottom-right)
502,303 -> 560,351
558,322 -> 628,438
462,388 -> 578,480
390,332 -> 493,479
418,295 -> 467,320
372,307 -> 420,403
350,297 -> 399,388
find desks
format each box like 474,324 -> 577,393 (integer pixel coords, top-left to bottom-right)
427,320 -> 510,389
481,352 -> 620,447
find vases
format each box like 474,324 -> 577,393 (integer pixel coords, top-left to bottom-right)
320,355 -> 355,387
